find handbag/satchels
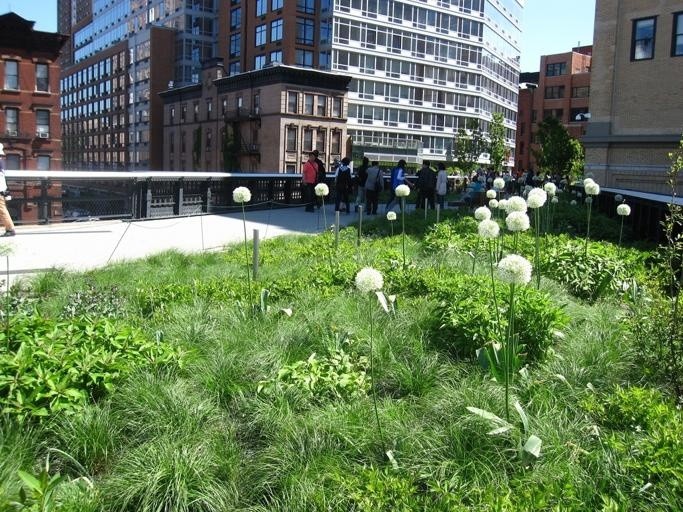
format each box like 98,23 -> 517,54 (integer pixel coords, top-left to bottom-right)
374,169 -> 383,192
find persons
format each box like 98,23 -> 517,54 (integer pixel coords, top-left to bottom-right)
0,172 -> 16,238
413,158 -> 571,209
364,160 -> 384,215
384,158 -> 407,213
354,156 -> 370,214
0,143 -> 6,172
333,157 -> 352,214
312,149 -> 326,208
302,151 -> 319,213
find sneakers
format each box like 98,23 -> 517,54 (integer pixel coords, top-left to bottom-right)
335,210 -> 358,215
305,205 -> 321,213
367,211 -> 377,215
0,230 -> 16,237
